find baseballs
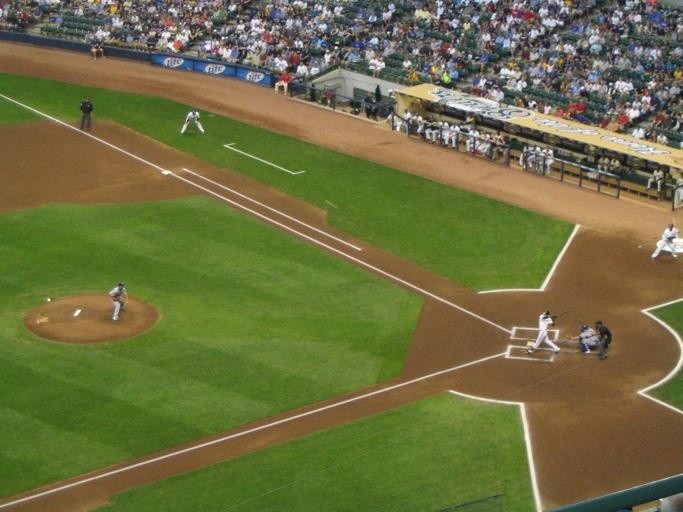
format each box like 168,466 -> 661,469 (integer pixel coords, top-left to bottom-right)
47,297 -> 51,302
637,246 -> 640,249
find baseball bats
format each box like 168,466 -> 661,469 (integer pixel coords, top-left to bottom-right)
557,309 -> 569,318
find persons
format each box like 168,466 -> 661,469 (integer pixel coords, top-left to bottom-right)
180,110 -> 205,134
526,309 -> 561,354
80,97 -> 93,131
108,281 -> 129,321
1,0 -> 309,96
650,222 -> 680,259
590,319 -> 612,361
564,324 -> 599,354
297,1 -> 682,195
